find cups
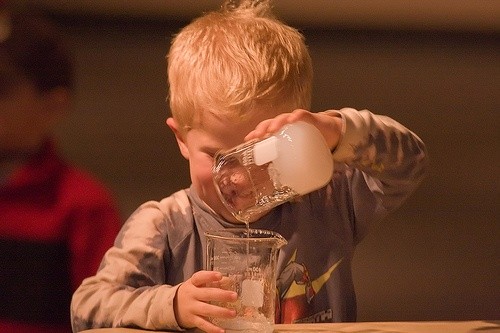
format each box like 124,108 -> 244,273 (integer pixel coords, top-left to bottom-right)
204,227 -> 288,333
212,121 -> 333,223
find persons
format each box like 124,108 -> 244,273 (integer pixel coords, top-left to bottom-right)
71,0 -> 431,332
